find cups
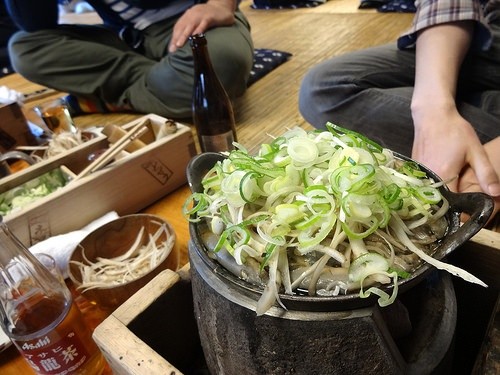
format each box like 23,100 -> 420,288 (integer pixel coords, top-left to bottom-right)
33,97 -> 78,134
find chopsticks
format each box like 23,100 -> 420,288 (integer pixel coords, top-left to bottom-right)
66,117 -> 150,191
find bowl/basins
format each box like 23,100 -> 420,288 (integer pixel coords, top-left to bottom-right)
66,214 -> 179,315
30,130 -> 99,159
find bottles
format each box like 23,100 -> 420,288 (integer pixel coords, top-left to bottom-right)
0,221 -> 106,375
190,34 -> 239,153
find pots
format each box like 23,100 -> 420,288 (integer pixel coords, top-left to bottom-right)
187,149 -> 495,374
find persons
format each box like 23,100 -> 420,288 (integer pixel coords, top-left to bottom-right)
298,0 -> 500,227
6,0 -> 253,118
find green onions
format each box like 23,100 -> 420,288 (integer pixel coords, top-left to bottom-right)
183,124 -> 445,307
